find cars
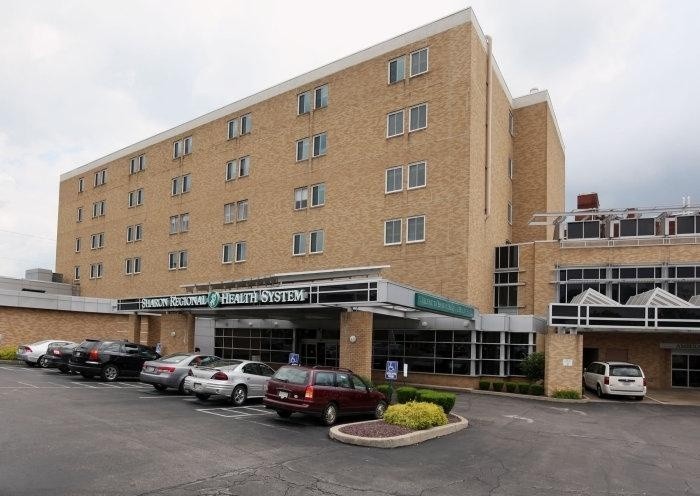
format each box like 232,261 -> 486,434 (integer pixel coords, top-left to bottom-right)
183,359 -> 276,407
582,361 -> 648,400
17,340 -> 81,375
139,352 -> 223,392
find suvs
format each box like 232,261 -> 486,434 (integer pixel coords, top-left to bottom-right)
70,338 -> 164,380
261,363 -> 388,426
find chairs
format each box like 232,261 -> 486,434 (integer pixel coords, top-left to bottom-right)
318,375 -> 331,386
